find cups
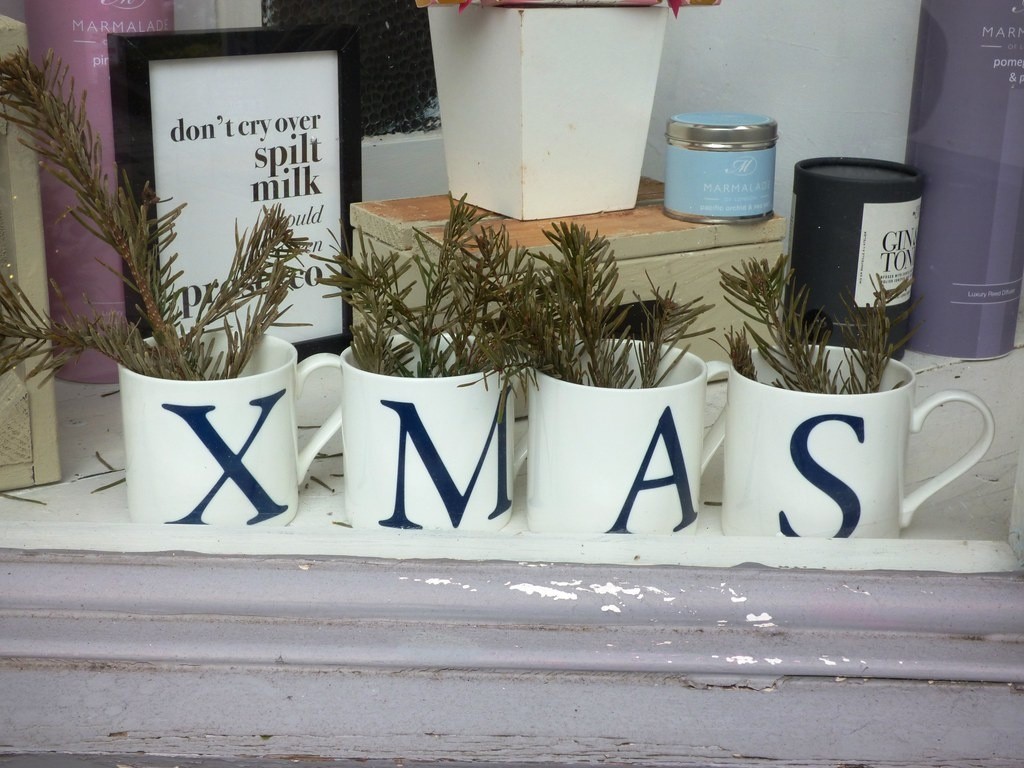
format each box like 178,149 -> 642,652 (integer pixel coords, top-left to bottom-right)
115,324 -> 344,526
337,334 -> 534,543
525,337 -> 733,533
722,344 -> 995,541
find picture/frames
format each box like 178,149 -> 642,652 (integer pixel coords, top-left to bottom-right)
108,28 -> 363,365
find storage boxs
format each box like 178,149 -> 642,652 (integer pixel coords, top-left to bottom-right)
351,174 -> 785,419
412,7 -> 683,221
